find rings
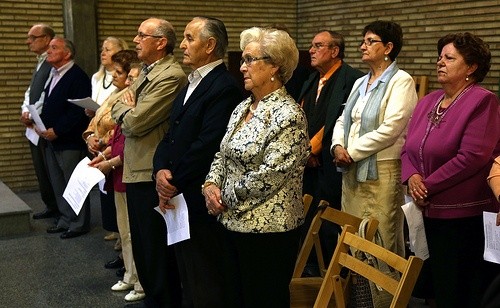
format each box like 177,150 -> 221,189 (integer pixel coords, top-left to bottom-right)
208,210 -> 212,215
413,189 -> 416,192
125,99 -> 128,102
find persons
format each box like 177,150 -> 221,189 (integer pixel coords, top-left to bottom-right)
399,33 -> 500,308
329,21 -> 418,308
21,25 -> 92,238
151,16 -> 253,308
201,27 -> 309,308
296,30 -> 367,275
83,18 -> 188,300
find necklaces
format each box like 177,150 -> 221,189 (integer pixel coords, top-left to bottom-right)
249,104 -> 256,114
434,82 -> 476,120
103,71 -> 113,89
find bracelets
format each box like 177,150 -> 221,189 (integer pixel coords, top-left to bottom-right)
86,134 -> 94,139
98,152 -> 106,160
202,182 -> 211,196
109,160 -> 115,170
331,144 -> 341,156
99,138 -> 106,147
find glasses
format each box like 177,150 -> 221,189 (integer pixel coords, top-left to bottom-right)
137,33 -> 162,41
308,43 -> 328,50
360,38 -> 383,46
239,55 -> 268,67
29,34 -> 45,41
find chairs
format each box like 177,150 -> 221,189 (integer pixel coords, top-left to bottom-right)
312,224 -> 425,308
288,200 -> 380,308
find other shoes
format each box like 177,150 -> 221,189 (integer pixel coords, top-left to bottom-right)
54,213 -> 61,225
33,207 -> 56,219
46,224 -> 67,233
59,230 -> 84,239
124,289 -> 146,301
114,237 -> 122,251
110,280 -> 133,291
115,268 -> 126,277
104,231 -> 119,240
104,256 -> 124,269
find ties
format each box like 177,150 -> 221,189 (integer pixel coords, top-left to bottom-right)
315,77 -> 328,104
